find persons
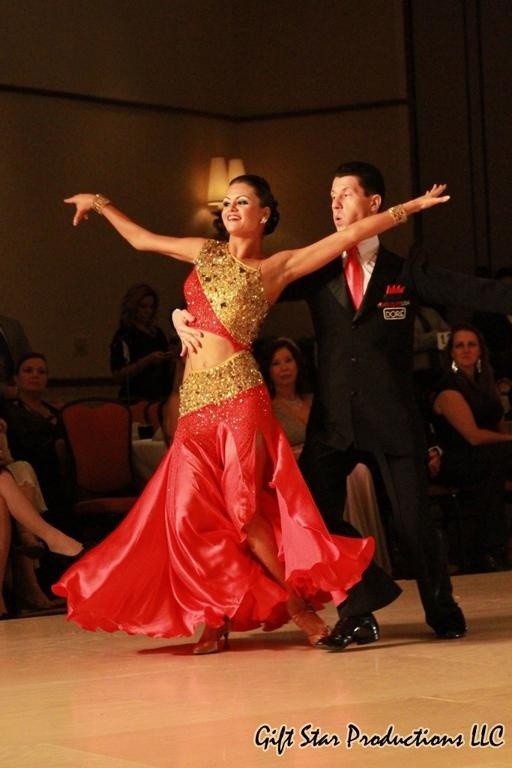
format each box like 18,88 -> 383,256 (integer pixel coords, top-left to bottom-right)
167,158 -> 469,650
2,281 -> 511,621
62,172 -> 453,654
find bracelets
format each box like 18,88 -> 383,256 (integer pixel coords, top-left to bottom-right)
91,191 -> 111,217
388,203 -> 409,226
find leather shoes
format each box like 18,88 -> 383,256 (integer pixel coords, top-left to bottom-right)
47,544 -> 89,564
318,614 -> 380,649
24,602 -> 51,608
426,604 -> 466,638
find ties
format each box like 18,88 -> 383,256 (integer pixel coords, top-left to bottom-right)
345,244 -> 364,311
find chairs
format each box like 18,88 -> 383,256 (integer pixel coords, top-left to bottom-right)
57,395 -> 175,523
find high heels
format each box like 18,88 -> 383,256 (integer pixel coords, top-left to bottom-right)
291,608 -> 331,645
194,617 -> 230,653
